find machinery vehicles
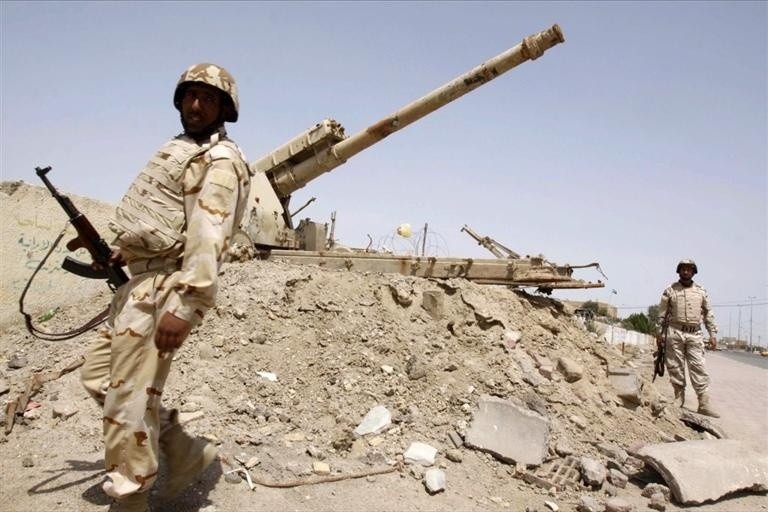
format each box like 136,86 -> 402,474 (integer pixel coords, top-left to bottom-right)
224,26 -> 608,292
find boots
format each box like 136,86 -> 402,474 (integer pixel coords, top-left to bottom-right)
158,425 -> 217,502
105,491 -> 149,512
672,384 -> 684,408
698,393 -> 720,418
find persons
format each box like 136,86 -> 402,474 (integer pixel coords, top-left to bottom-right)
79,64 -> 257,511
654,258 -> 721,418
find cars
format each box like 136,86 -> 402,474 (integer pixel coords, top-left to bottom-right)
705,342 -> 768,357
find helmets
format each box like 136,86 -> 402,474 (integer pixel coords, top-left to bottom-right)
676,259 -> 697,273
174,63 -> 239,123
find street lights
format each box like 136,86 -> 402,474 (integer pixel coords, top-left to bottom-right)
747,296 -> 757,351
736,303 -> 746,343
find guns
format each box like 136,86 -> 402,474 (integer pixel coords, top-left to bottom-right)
652,296 -> 674,383
35,166 -> 130,294
